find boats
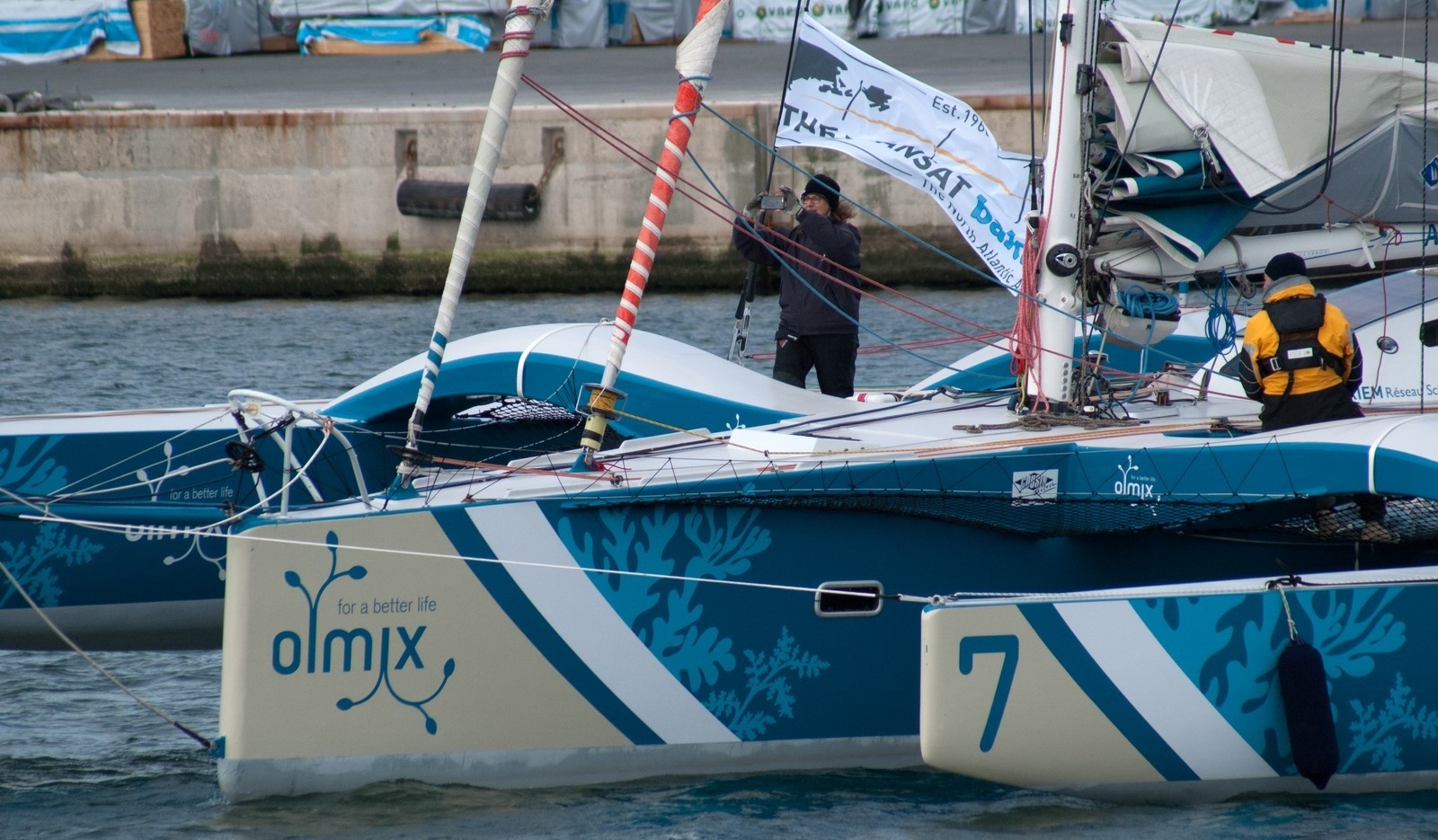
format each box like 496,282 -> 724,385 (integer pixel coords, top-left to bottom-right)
1,1 -> 1438,645
222,3 -> 1438,806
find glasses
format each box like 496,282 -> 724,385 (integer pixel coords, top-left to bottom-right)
801,195 -> 823,204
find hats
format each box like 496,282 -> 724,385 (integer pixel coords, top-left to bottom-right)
1264,252 -> 1307,281
803,174 -> 841,213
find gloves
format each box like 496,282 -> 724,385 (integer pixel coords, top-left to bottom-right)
742,191 -> 768,223
775,184 -> 802,216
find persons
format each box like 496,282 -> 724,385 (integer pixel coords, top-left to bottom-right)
1238,253 -> 1366,432
731,174 -> 863,400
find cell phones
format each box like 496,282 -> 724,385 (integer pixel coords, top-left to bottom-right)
760,195 -> 785,210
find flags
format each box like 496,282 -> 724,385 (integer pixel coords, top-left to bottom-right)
773,10 -> 1044,297
